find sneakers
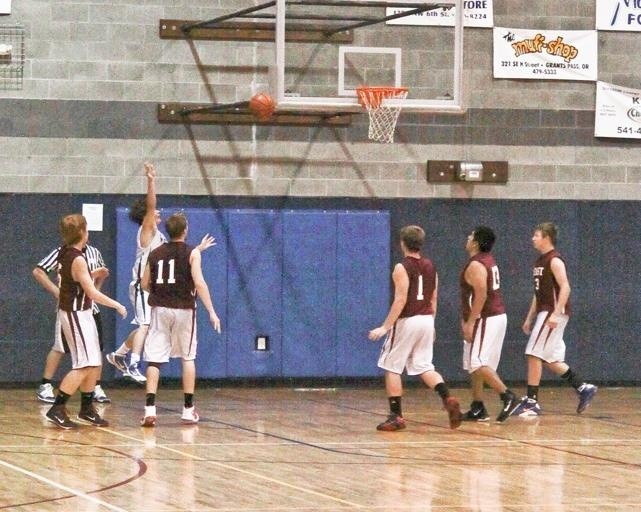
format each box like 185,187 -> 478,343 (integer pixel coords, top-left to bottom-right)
513,398 -> 543,416
139,405 -> 158,427
576,384 -> 598,413
106,351 -> 128,374
94,385 -> 111,402
44,407 -> 78,430
462,403 -> 490,423
37,383 -> 56,403
180,406 -> 200,424
77,408 -> 110,427
496,393 -> 522,423
446,397 -> 462,429
123,367 -> 147,383
375,416 -> 408,431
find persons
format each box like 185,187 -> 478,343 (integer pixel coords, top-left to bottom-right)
45,214 -> 127,430
368,225 -> 462,430
460,225 -> 525,425
510,223 -> 599,417
141,213 -> 221,427
32,235 -> 112,403
105,160 -> 217,385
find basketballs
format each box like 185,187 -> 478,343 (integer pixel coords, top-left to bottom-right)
249,94 -> 277,121
